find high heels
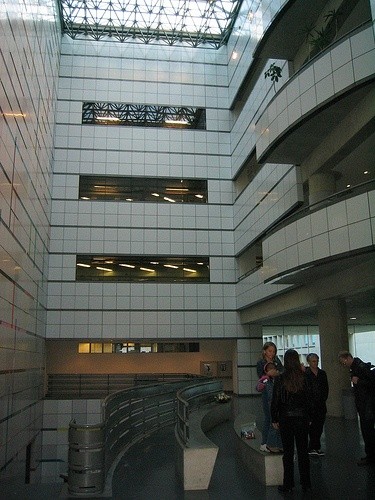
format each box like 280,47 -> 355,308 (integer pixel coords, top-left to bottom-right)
266,447 -> 283,455
302,486 -> 312,497
278,486 -> 292,497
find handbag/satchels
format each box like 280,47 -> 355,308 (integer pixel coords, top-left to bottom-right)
255,369 -> 275,391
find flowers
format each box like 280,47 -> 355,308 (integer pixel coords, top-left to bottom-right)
213,392 -> 232,401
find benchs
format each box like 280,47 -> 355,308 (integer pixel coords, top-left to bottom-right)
175,380 -> 234,490
233,412 -> 285,487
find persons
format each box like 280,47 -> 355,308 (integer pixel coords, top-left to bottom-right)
257,341 -> 284,453
256,362 -> 284,453
305,353 -> 328,455
270,348 -> 312,495
337,351 -> 375,466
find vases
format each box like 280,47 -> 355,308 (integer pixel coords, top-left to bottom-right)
218,400 -> 229,404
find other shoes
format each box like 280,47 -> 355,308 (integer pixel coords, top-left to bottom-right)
309,448 -> 325,456
259,444 -> 270,452
361,457 -> 369,463
